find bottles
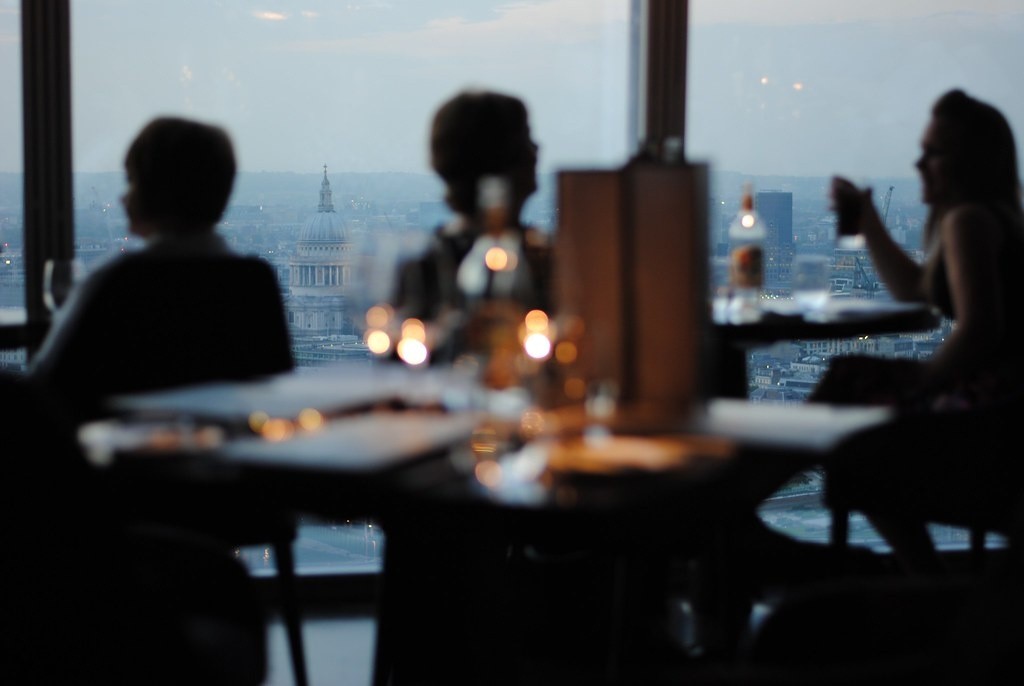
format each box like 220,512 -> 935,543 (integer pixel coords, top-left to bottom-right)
729,184 -> 767,308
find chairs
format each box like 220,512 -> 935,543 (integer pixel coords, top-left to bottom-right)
0,249 -> 307,685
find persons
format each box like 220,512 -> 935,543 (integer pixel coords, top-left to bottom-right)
808,91 -> 1024,550
30,117 -> 296,420
390,94 -> 548,365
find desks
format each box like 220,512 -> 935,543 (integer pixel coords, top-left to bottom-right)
714,301 -> 943,404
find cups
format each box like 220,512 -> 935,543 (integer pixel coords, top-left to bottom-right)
793,256 -> 832,309
839,234 -> 865,250
